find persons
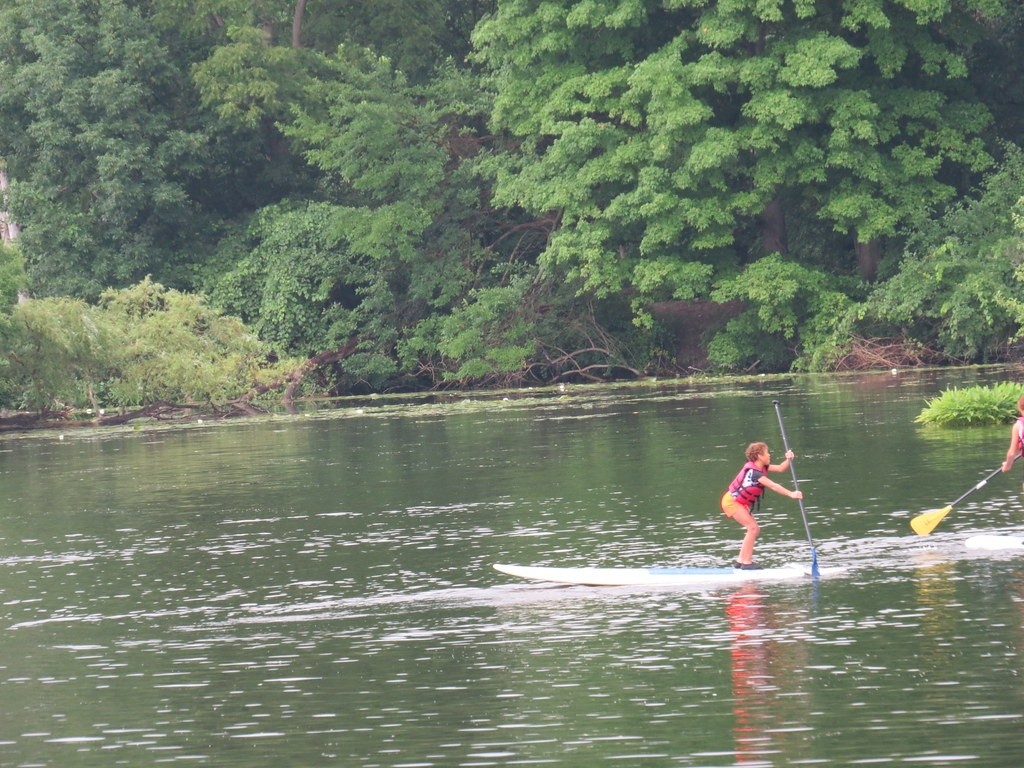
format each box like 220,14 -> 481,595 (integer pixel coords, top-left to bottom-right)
721,442 -> 803,570
1001,394 -> 1024,472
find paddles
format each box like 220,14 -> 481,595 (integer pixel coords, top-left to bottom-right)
770,400 -> 819,576
910,453 -> 1022,535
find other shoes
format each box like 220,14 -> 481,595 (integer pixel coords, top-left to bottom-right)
741,562 -> 763,570
734,562 -> 741,568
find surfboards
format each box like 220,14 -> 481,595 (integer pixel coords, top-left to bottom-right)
492,561 -> 850,587
